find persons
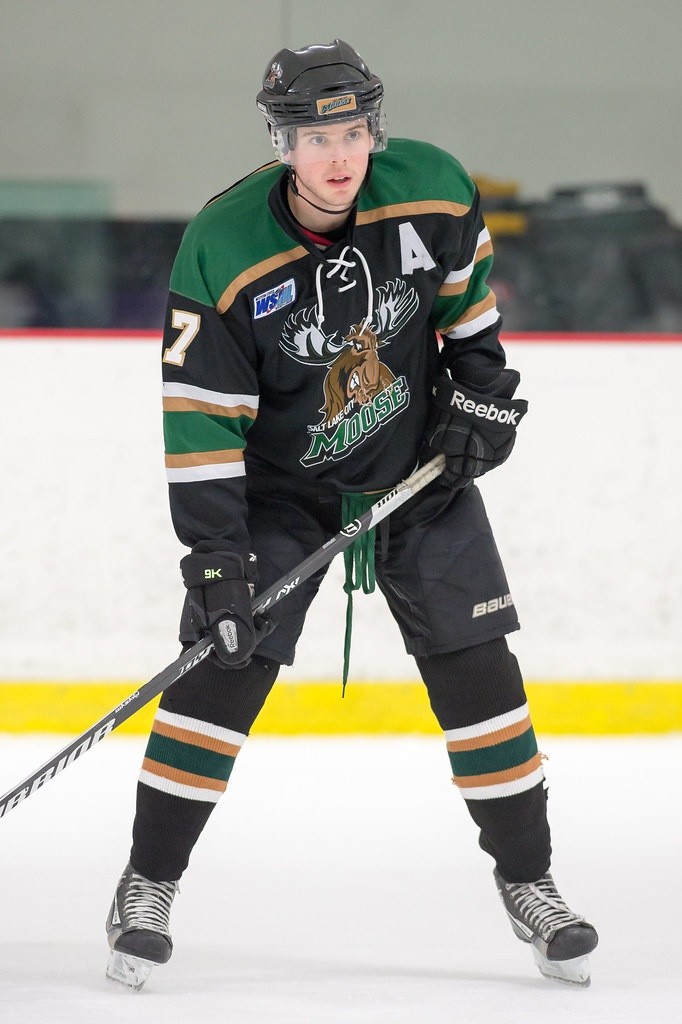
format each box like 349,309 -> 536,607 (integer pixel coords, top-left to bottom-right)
104,40 -> 600,987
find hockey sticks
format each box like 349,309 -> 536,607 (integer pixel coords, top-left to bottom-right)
0,455 -> 448,818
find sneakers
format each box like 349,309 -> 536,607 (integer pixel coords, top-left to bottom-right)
105,859 -> 180,994
493,866 -> 599,991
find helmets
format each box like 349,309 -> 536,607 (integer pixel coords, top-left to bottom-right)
256,38 -> 388,165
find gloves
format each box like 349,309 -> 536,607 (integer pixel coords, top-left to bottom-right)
178,539 -> 279,670
422,346 -> 527,489
